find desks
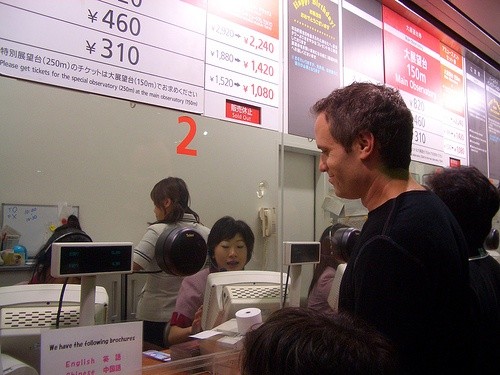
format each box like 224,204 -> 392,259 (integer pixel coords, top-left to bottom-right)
143,336 -> 240,375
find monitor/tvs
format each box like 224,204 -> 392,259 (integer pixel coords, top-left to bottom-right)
202,270 -> 291,330
0,284 -> 109,375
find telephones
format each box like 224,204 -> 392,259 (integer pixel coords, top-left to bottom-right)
261,207 -> 279,238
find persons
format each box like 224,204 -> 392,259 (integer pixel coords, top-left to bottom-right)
167,215 -> 255,346
302,223 -> 357,330
307,82 -> 477,375
126,177 -> 213,350
425,162 -> 500,375
241,305 -> 386,375
15,215 -> 100,284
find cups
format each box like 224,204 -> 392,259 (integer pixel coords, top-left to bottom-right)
14,246 -> 25,264
19,245 -> 28,261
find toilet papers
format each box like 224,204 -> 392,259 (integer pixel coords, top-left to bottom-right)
235,306 -> 265,335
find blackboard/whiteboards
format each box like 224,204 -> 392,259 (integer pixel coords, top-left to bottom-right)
3,203 -> 80,261
